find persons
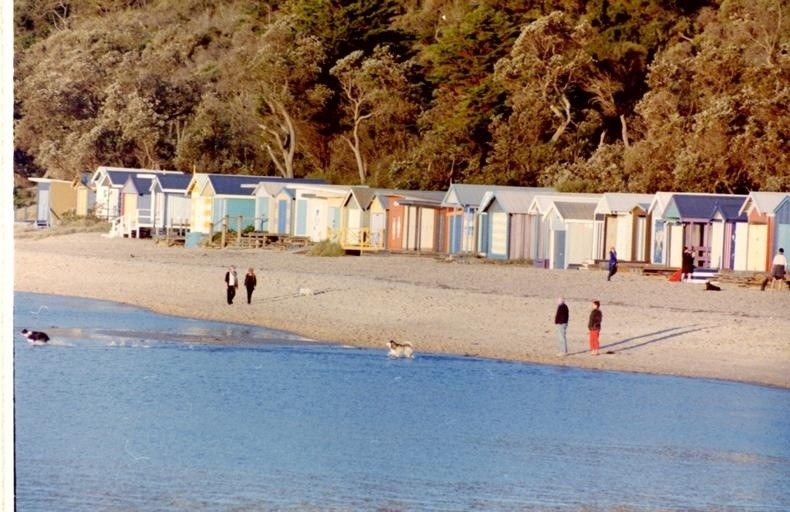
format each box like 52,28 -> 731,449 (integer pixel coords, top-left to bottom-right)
683,246 -> 694,280
244,268 -> 257,304
607,247 -> 617,281
587,301 -> 603,354
554,296 -> 569,355
21,328 -> 50,345
225,265 -> 239,305
771,248 -> 787,293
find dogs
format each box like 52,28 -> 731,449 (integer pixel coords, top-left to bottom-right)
299,286 -> 314,296
703,280 -> 721,291
386,340 -> 415,359
19,329 -> 50,343
